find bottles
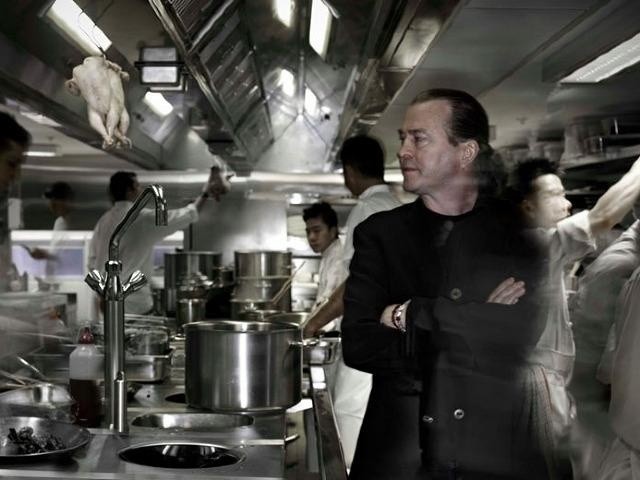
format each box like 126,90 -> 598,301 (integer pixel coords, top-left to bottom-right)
67,326 -> 106,428
41,315 -> 65,352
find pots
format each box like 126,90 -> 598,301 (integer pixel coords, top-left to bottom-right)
181,319 -> 320,413
164,250 -> 297,319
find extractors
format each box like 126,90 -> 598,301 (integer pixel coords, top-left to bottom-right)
0,1 -> 468,174
285,186 -> 359,237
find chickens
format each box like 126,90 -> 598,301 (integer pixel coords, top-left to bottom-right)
64,54 -> 134,152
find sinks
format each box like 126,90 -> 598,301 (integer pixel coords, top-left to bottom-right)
131,410 -> 256,430
117,443 -> 247,470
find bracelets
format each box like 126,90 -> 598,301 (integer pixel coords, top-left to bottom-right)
391,304 -> 401,331
198,191 -> 209,199
394,300 -> 410,333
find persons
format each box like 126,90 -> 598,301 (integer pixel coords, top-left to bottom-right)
512,154 -> 640,447
303,135 -> 404,474
341,86 -> 564,480
86,169 -> 223,324
591,266 -> 640,480
564,192 -> 640,480
26,181 -> 76,283
303,200 -> 346,398
1,110 -> 30,194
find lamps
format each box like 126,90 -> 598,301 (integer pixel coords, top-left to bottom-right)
23,144 -> 57,158
541,1 -> 640,88
133,42 -> 193,94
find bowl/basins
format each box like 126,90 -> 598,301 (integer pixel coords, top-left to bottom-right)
0,416 -> 91,459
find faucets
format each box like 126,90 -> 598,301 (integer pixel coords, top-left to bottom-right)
82,183 -> 169,431
